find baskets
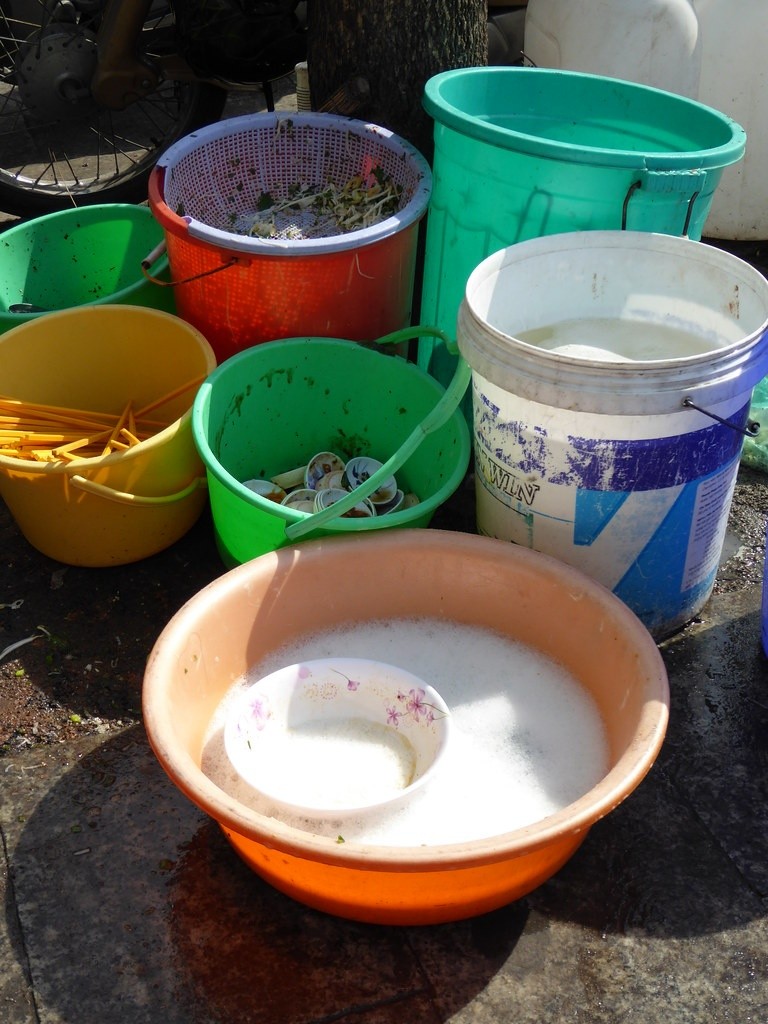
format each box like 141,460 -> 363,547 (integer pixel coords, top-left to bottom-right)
156,111 -> 433,256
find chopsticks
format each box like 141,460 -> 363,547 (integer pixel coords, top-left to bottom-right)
0,375 -> 205,462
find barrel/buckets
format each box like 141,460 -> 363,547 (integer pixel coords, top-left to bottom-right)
0,304 -> 223,569
1,205 -> 174,356
419,62 -> 748,383
191,326 -> 470,574
149,110 -> 432,370
459,230 -> 767,643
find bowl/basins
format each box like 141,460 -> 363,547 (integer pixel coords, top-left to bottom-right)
242,452 -> 404,519
224,655 -> 450,819
139,529 -> 673,924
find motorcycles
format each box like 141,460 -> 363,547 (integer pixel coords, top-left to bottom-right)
0,1 -> 526,219
